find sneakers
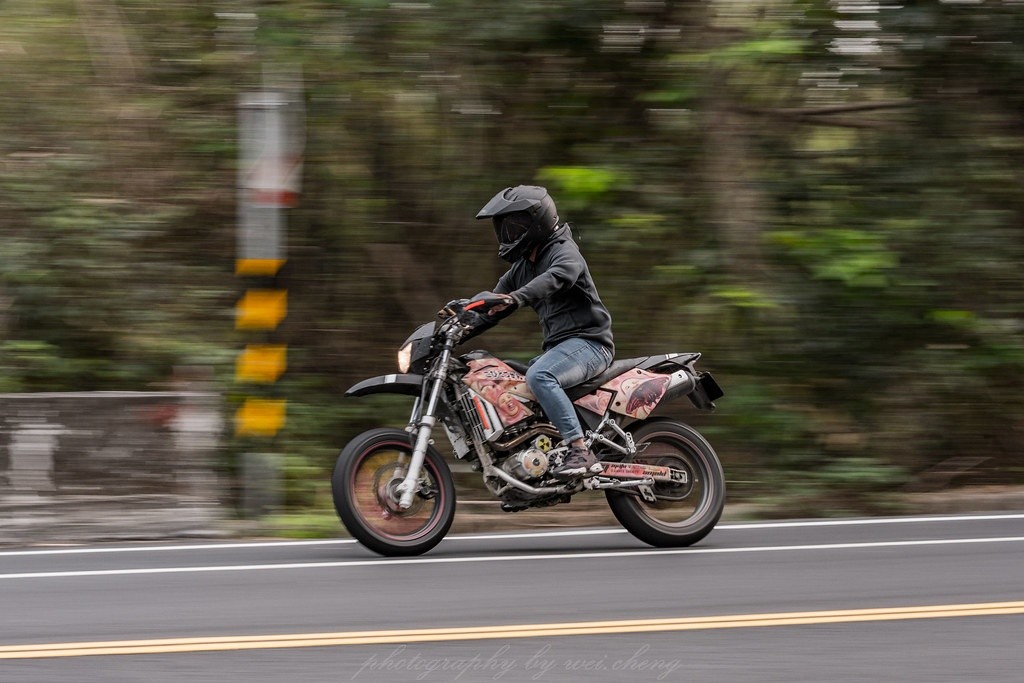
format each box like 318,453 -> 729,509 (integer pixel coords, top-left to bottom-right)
553,447 -> 603,479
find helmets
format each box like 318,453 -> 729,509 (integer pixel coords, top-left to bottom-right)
476,185 -> 559,263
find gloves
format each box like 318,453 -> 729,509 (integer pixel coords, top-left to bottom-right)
470,291 -> 515,317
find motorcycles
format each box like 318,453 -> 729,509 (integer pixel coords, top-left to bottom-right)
333,292 -> 727,557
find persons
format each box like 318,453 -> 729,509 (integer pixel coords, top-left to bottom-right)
438,184 -> 615,513
